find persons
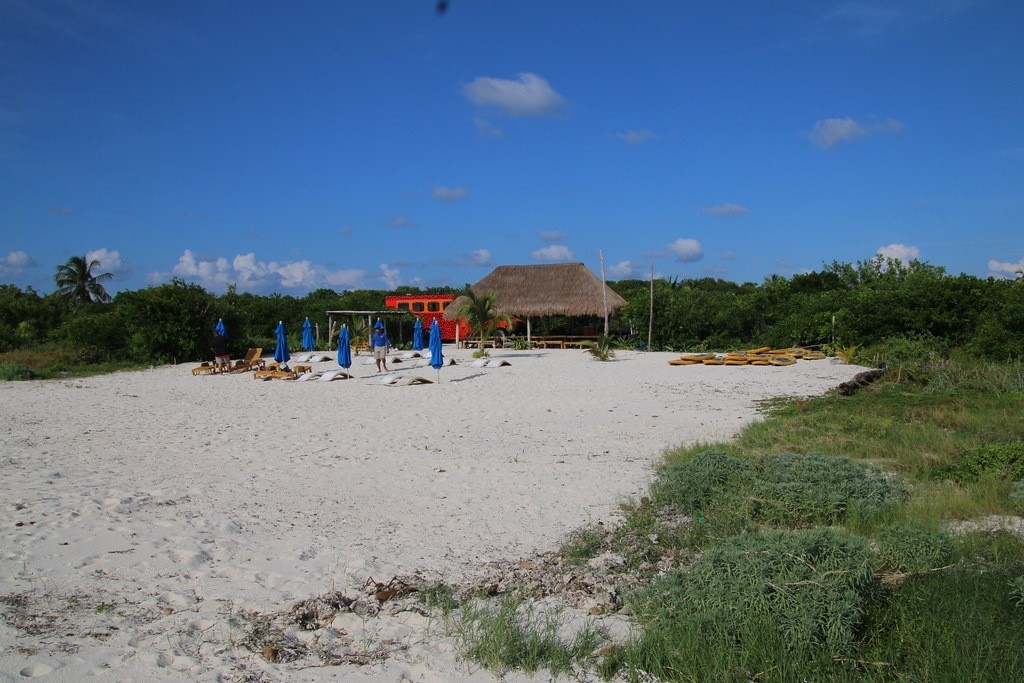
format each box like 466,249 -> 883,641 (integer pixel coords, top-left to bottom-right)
209,331 -> 231,376
371,327 -> 390,373
258,363 -> 292,372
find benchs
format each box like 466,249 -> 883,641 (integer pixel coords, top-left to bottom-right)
462,338 -> 598,351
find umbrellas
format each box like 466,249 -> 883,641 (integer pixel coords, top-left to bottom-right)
302,317 -> 314,352
274,321 -> 291,363
374,317 -> 384,329
215,318 -> 225,336
413,318 -> 424,352
429,317 -> 444,384
338,324 -> 352,380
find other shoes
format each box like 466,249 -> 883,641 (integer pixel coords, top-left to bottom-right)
383,369 -> 388,371
376,370 -> 381,373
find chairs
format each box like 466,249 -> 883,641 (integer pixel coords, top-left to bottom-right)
192,348 -> 312,380
